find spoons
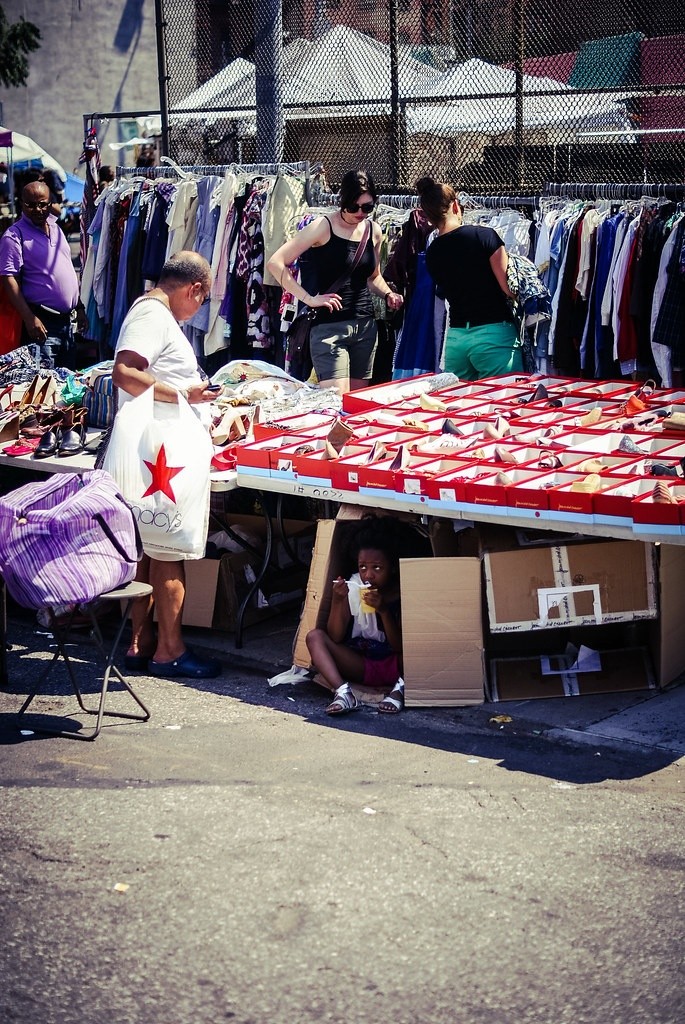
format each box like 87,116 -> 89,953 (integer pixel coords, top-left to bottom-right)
333,580 -> 372,588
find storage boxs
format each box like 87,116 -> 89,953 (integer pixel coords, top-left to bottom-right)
122,491 -> 685,707
236,372 -> 685,535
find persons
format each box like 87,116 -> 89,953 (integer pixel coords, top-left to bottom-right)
416,177 -> 524,380
305,536 -> 404,714
0,180 -> 79,368
110,250 -> 224,678
265,169 -> 404,394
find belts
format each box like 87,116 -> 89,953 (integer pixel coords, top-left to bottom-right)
451,317 -> 511,329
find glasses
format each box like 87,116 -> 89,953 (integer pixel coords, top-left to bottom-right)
454,198 -> 465,214
192,282 -> 212,306
346,203 -> 376,214
21,200 -> 51,208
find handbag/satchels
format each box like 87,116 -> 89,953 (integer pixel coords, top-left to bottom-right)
101,384 -> 211,562
1,469 -> 144,629
287,306 -> 313,356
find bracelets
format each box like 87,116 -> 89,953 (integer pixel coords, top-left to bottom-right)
385,292 -> 390,300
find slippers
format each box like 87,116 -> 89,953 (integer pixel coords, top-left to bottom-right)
148,649 -> 223,679
125,643 -> 207,671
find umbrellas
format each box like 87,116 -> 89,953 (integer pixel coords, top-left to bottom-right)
138,27 -> 626,181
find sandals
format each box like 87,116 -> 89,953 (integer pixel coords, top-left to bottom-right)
379,676 -> 404,715
327,682 -> 363,714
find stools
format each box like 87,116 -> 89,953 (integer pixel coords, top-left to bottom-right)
13,580 -> 151,740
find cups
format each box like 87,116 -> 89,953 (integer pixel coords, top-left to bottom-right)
359,589 -> 378,613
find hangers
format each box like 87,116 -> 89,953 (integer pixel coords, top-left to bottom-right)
93,156 -> 324,206
535,182 -> 685,230
306,192 -> 526,230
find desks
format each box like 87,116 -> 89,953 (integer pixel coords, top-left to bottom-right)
0,380 -> 288,580
235,474 -> 684,649
